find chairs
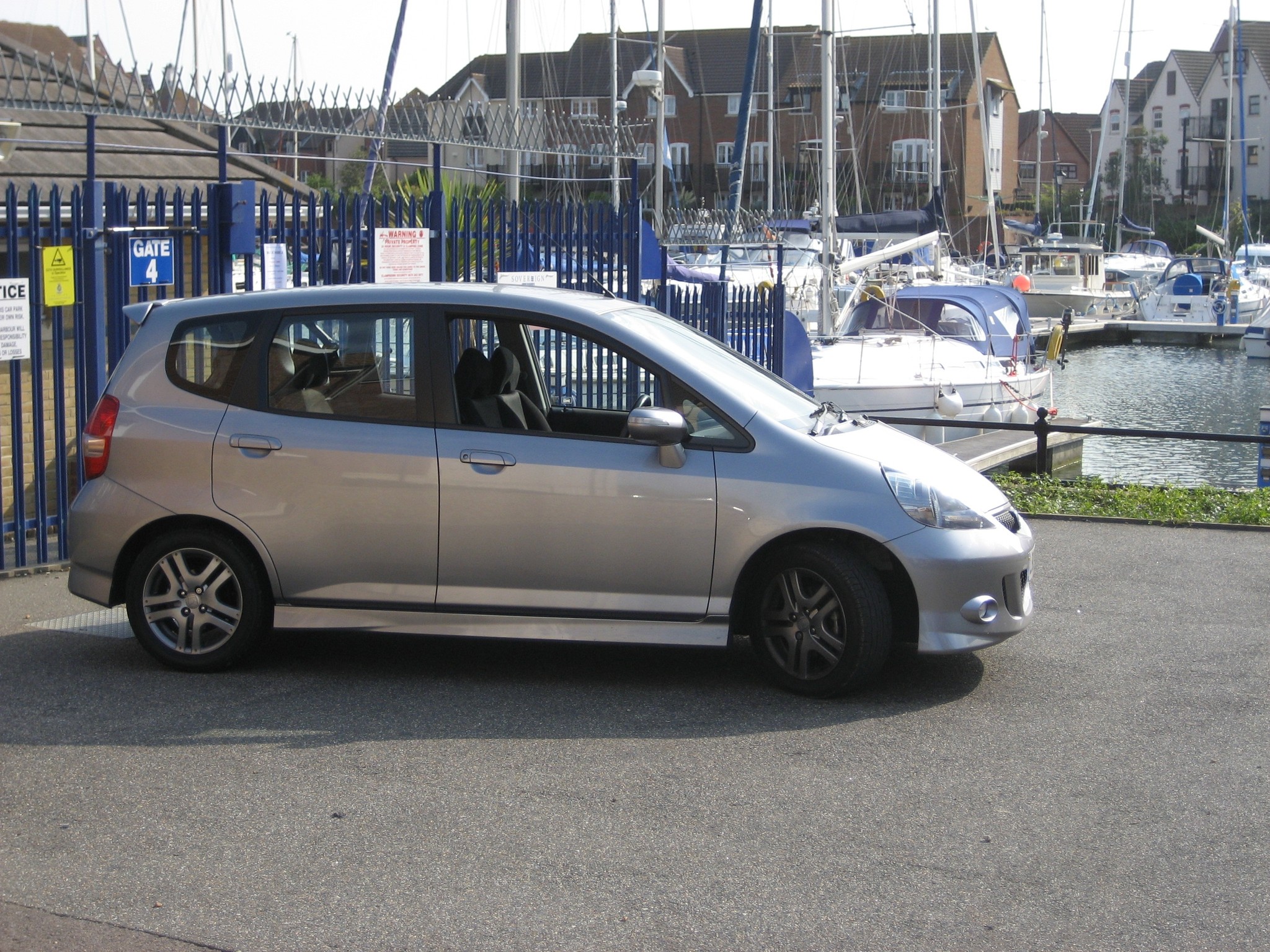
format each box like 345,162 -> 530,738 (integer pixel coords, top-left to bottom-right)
455,348 -> 526,432
490,346 -> 553,432
268,326 -> 333,414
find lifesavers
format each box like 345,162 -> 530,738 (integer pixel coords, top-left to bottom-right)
978,241 -> 994,254
1047,324 -> 1064,360
694,245 -> 707,252
1227,280 -> 1240,298
861,285 -> 884,302
758,281 -> 774,303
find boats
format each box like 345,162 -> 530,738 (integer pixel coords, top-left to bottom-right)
814,282 -> 1055,441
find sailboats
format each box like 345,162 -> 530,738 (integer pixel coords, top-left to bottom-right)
341,0 -> 1173,405
1138,0 -> 1270,359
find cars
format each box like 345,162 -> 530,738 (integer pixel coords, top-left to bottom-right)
68,280 -> 1033,697
